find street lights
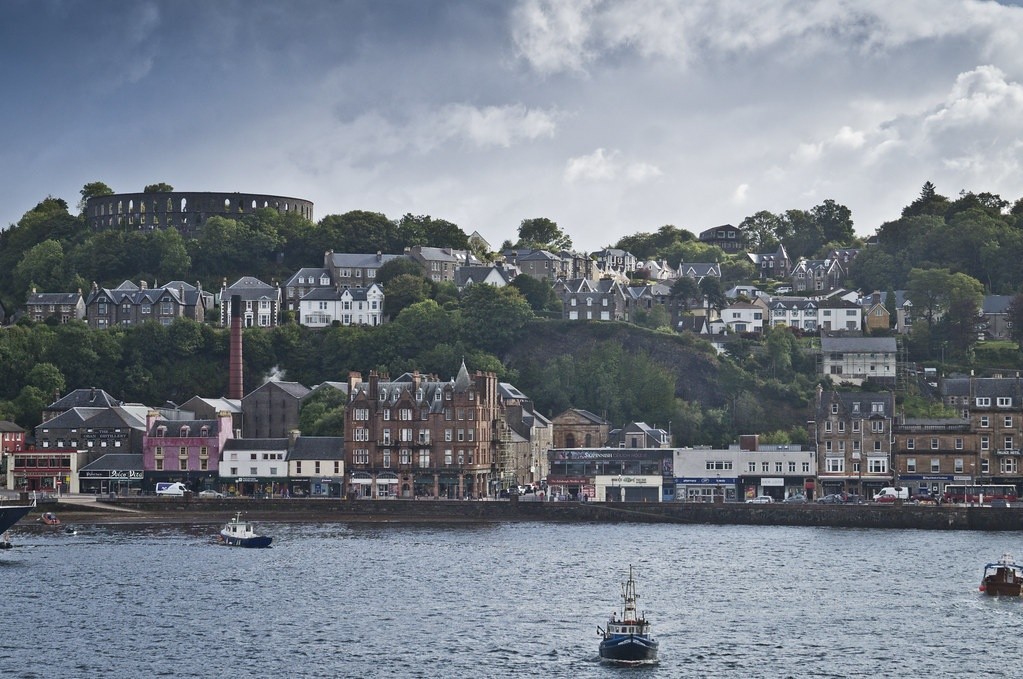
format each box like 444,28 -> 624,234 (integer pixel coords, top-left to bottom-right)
807,420 -> 819,500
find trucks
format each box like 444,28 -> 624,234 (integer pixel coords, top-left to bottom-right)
155,482 -> 192,496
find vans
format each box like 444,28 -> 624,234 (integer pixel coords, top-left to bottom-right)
873,487 -> 909,502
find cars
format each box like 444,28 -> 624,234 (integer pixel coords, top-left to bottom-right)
782,494 -> 808,504
199,489 -> 225,498
817,494 -> 844,504
876,494 -> 896,503
744,495 -> 774,504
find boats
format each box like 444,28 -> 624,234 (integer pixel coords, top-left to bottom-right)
596,564 -> 660,661
979,553 -> 1023,596
220,511 -> 273,547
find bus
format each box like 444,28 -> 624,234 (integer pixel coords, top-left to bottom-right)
945,484 -> 1018,504
